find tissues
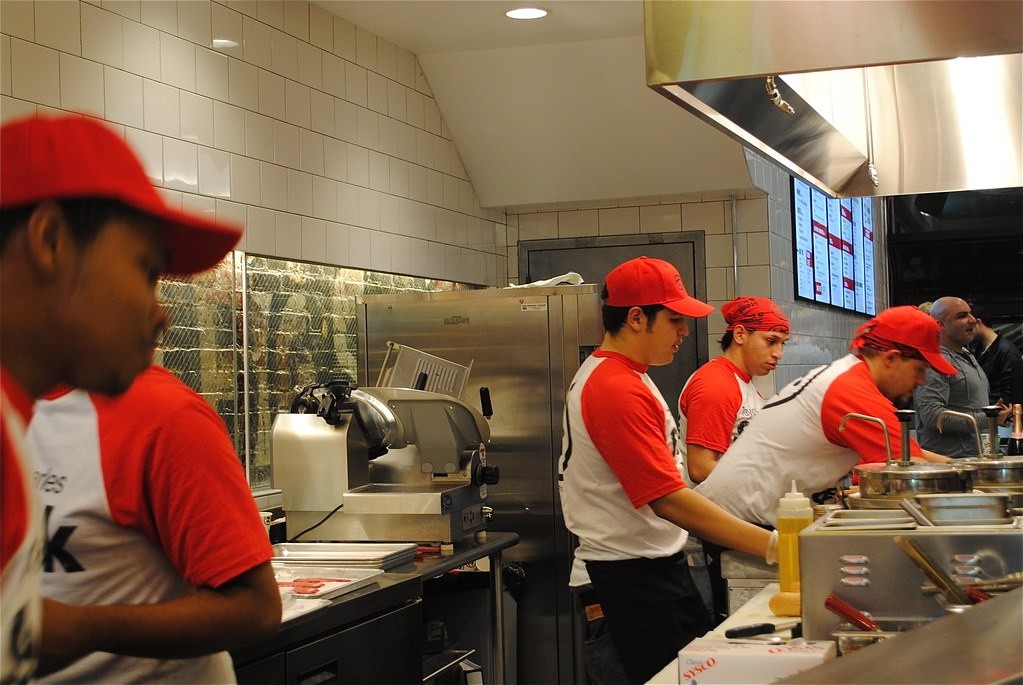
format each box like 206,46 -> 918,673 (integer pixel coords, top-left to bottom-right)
677,636 -> 837,684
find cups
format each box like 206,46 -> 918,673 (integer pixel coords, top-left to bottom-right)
260,512 -> 273,537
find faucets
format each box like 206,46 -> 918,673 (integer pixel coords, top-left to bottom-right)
937,410 -> 985,458
838,412 -> 896,465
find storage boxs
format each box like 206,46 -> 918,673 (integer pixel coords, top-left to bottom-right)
678,637 -> 836,685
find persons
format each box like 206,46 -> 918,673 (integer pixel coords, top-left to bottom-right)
559,256 -> 778,684
28,364 -> 281,685
967,299 -> 1023,410
0,114 -> 241,685
914,297 -> 1012,458
677,297 -> 789,489
685,305 -> 954,623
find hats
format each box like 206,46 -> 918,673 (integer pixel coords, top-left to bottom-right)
602,256 -> 715,318
1,117 -> 241,276
858,305 -> 959,378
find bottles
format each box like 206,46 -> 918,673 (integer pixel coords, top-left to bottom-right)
776,480 -> 814,593
1007,404 -> 1023,456
